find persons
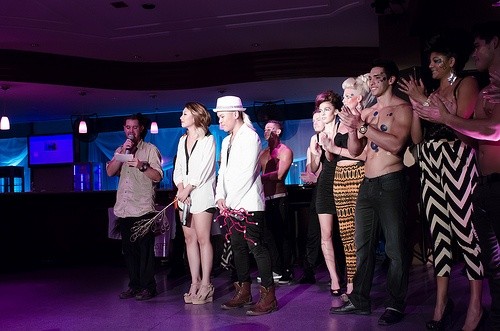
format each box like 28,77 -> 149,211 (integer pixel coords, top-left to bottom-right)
257,120 -> 295,283
173,99 -> 215,306
105,113 -> 164,302
212,96 -> 279,317
297,23 -> 500,331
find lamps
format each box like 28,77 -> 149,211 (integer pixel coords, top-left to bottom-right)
79,95 -> 88,134
151,95 -> 158,134
0,84 -> 12,130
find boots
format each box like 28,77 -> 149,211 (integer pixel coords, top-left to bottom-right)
220,281 -> 252,309
245,285 -> 278,316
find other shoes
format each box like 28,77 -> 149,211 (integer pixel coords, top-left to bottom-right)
135,289 -> 158,300
119,288 -> 141,299
256,270 -> 291,284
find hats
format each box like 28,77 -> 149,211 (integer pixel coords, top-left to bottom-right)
213,95 -> 247,112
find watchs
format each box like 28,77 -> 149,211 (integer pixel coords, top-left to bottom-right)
192,185 -> 196,189
422,98 -> 432,107
359,123 -> 369,134
139,161 -> 150,172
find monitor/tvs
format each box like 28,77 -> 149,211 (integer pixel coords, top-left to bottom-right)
27,132 -> 77,167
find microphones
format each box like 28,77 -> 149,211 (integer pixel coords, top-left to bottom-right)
126,135 -> 134,154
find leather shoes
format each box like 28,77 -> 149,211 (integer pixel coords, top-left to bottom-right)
378,303 -> 406,326
329,300 -> 372,315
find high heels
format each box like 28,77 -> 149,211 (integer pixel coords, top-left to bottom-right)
461,309 -> 488,331
184,281 -> 202,304
345,289 -> 353,299
426,298 -> 453,331
192,283 -> 215,305
330,278 -> 341,296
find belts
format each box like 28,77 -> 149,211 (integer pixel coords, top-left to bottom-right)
265,191 -> 288,201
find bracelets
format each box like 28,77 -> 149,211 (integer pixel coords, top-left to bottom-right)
338,148 -> 343,157
